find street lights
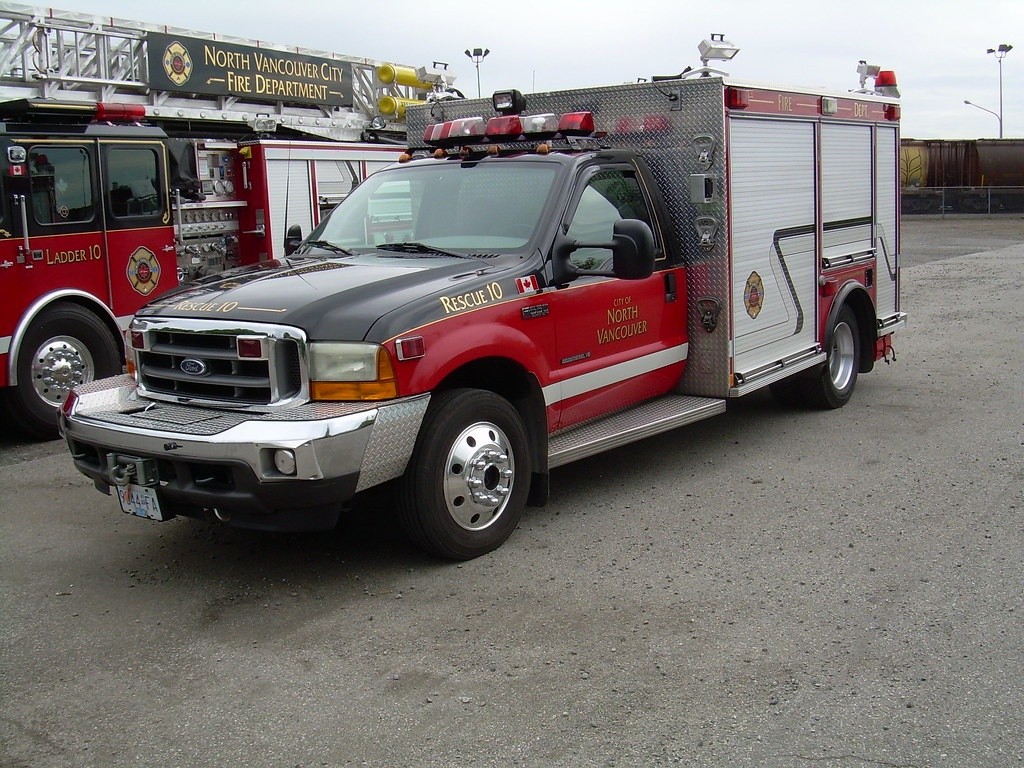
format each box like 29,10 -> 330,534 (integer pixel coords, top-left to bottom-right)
465,49 -> 490,99
964,44 -> 1013,138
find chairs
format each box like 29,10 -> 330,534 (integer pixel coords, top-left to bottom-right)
568,181 -> 634,267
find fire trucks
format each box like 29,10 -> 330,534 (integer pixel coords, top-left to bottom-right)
56,33 -> 908,561
0,2 -> 467,440
